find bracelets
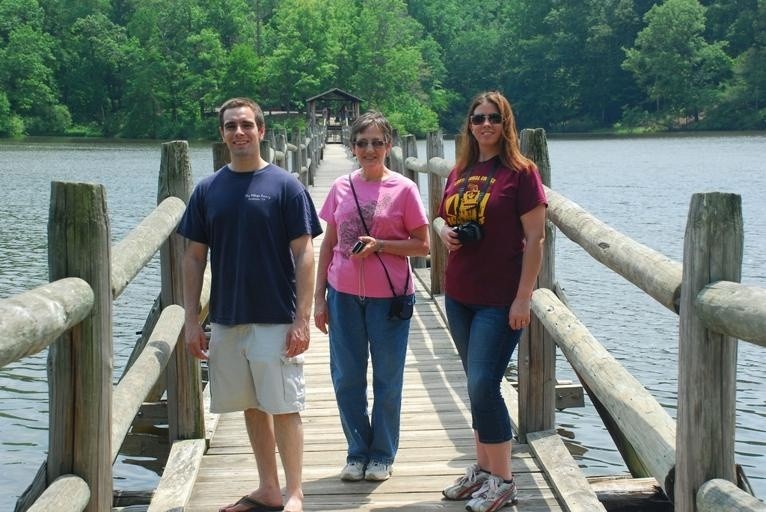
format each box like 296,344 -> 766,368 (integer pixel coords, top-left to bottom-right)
378,240 -> 385,253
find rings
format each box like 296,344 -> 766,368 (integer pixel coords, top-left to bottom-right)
522,320 -> 526,326
302,347 -> 305,351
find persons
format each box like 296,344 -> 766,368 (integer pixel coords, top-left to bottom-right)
432,90 -> 549,511
312,111 -> 431,482
175,97 -> 323,511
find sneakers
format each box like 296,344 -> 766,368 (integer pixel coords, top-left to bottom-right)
341,462 -> 367,479
441,466 -> 490,500
466,474 -> 517,511
365,458 -> 393,481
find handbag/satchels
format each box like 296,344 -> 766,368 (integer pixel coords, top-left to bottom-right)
390,295 -> 414,322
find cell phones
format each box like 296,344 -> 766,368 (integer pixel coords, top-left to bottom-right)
351,242 -> 366,254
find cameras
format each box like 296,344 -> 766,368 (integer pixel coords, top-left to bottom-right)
454,222 -> 485,246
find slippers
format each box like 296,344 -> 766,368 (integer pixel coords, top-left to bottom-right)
219,493 -> 285,512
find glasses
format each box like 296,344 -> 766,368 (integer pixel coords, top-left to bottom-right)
472,113 -> 503,125
357,140 -> 384,149
